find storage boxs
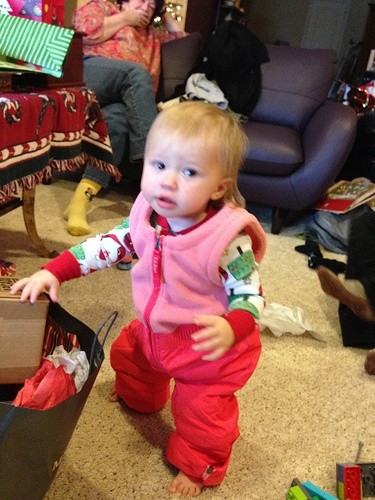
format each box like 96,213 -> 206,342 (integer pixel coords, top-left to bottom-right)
0,259 -> 52,385
0,0 -> 86,94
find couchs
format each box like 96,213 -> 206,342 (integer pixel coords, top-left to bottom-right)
150,32 -> 358,235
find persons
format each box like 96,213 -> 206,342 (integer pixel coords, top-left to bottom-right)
318,208 -> 374,351
70,0 -> 189,236
10,100 -> 268,497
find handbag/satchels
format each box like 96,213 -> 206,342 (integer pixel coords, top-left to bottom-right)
0,293 -> 118,500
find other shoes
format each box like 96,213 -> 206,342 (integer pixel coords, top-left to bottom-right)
364,349 -> 375,374
318,265 -> 374,322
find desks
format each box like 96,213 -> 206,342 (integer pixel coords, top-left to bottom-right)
0,87 -> 114,259
